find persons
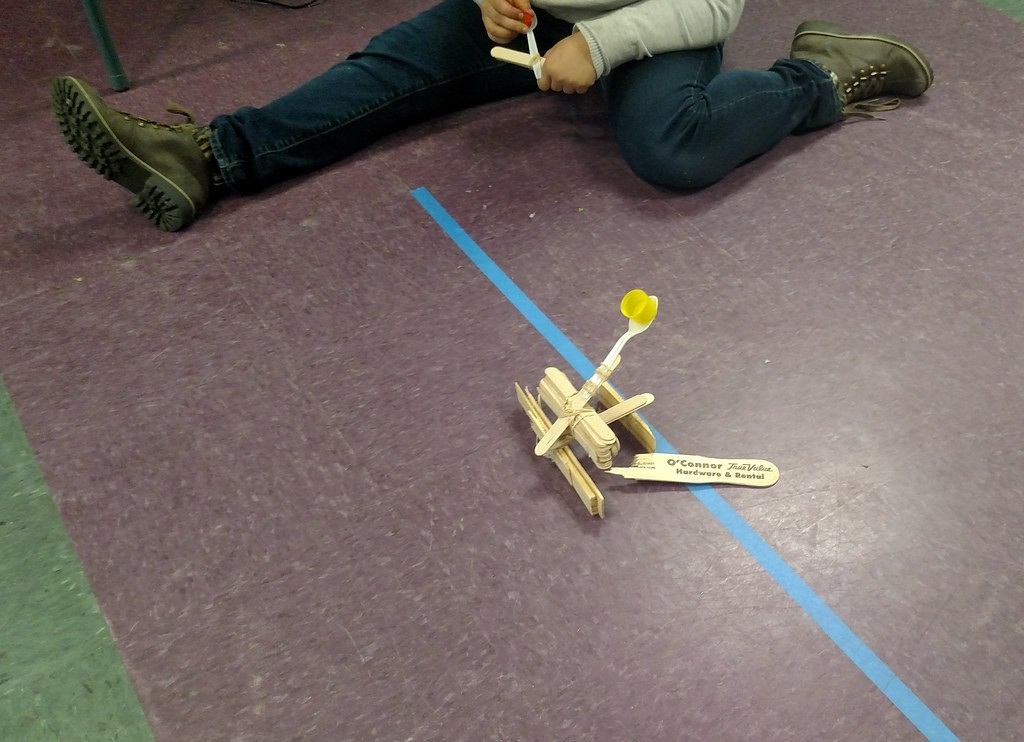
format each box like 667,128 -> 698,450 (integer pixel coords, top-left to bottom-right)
50,0 -> 934,232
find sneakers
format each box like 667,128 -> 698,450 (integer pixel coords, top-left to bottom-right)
53,76 -> 225,231
789,21 -> 934,120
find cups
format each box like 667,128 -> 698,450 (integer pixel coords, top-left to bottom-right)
620,290 -> 657,325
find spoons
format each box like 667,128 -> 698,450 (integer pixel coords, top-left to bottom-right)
524,16 -> 542,80
582,296 -> 659,398
